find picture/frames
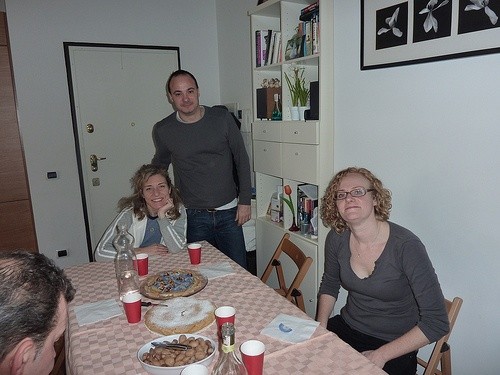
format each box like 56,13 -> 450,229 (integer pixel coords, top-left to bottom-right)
360,0 -> 500,71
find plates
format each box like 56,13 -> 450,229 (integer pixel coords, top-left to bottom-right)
144,298 -> 219,337
140,268 -> 208,300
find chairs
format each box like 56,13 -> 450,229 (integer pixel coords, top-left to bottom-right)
259,233 -> 313,314
416,295 -> 463,375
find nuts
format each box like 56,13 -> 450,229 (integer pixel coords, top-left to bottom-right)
143,335 -> 214,367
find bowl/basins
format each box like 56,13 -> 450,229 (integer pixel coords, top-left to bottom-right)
137,334 -> 217,375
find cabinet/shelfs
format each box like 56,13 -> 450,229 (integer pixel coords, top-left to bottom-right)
248,0 -> 334,321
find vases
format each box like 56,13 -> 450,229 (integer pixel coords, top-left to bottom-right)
288,216 -> 300,232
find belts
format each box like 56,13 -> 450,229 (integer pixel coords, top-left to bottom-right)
195,208 -> 226,213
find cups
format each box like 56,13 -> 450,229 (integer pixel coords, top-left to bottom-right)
239,340 -> 265,375
215,306 -> 236,340
133,253 -> 148,276
121,294 -> 142,324
188,243 -> 202,264
180,364 -> 209,375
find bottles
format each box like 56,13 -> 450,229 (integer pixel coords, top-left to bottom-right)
210,322 -> 248,375
112,221 -> 141,300
270,185 -> 283,223
271,93 -> 282,121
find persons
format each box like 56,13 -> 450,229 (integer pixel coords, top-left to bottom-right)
95,164 -> 187,262
0,250 -> 76,375
316,168 -> 450,375
153,70 -> 252,268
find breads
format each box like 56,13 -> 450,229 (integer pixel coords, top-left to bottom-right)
144,296 -> 219,335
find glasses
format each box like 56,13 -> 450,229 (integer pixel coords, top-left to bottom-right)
334,187 -> 377,200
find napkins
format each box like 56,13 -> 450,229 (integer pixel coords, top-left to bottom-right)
73,298 -> 125,328
198,260 -> 237,280
259,312 -> 321,344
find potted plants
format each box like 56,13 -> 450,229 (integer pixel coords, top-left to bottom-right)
282,64 -> 310,122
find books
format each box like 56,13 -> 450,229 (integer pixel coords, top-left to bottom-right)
297,183 -> 317,230
297,1 -> 319,57
255,30 -> 281,68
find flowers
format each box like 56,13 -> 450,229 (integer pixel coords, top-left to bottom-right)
280,185 -> 296,217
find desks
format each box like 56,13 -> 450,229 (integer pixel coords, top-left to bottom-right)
61,240 -> 391,375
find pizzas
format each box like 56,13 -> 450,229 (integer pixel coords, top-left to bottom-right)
144,269 -> 205,298
